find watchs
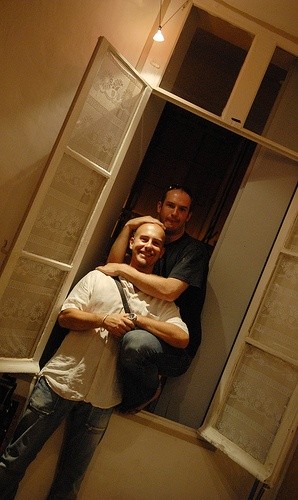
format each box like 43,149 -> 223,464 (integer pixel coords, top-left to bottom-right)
127,312 -> 138,328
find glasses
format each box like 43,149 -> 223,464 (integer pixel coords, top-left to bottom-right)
162,182 -> 195,197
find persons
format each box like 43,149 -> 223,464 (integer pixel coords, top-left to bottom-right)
93,183 -> 210,417
0,221 -> 191,500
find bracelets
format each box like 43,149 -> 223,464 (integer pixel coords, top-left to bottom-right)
100,314 -> 109,332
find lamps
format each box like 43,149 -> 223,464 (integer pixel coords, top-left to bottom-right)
153,1 -> 193,42
